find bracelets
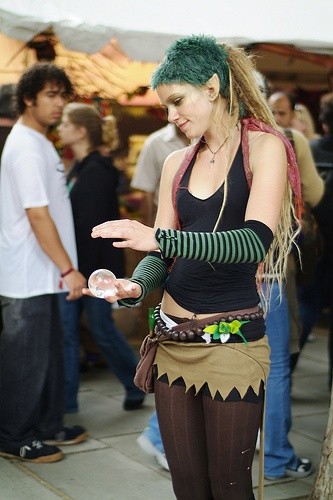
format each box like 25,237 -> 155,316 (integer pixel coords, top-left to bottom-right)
59,267 -> 74,289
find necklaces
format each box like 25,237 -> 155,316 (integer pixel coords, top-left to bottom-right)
205,119 -> 239,163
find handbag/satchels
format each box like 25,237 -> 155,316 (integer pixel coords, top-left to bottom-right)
296,202 -> 320,291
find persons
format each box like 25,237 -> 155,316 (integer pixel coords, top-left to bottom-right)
252,70 -> 324,481
82,32 -> 303,500
136,91 -> 333,471
0,62 -> 89,464
0,83 -> 20,165
56,102 -> 146,414
129,122 -> 191,301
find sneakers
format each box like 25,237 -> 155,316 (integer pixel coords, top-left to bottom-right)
1,420 -> 88,463
135,432 -> 169,472
264,458 -> 312,480
122,399 -> 143,410
